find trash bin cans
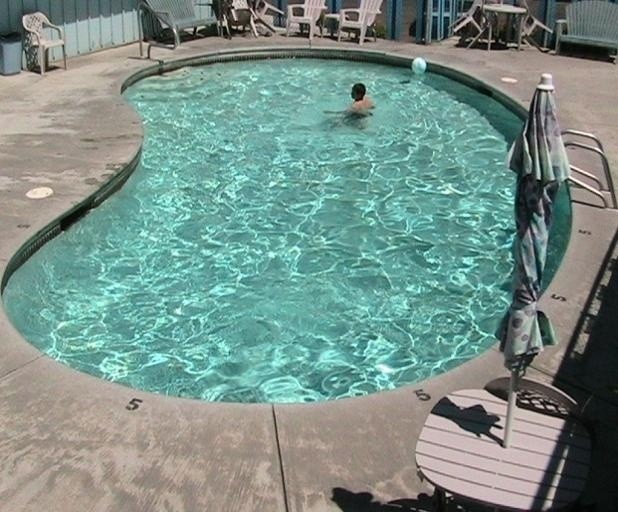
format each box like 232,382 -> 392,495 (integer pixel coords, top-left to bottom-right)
0,31 -> 23,76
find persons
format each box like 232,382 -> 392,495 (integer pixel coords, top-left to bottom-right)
345,83 -> 376,118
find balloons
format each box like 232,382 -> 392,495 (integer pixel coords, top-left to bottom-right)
411,55 -> 426,75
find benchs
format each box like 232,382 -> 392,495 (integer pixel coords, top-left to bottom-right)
141,0 -> 220,46
553,0 -> 618,63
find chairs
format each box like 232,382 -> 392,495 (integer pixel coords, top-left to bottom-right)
485,377 -> 581,426
449,1 -> 553,52
21,11 -> 68,75
219,0 -> 383,46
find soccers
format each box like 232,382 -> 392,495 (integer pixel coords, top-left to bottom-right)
413,57 -> 426,74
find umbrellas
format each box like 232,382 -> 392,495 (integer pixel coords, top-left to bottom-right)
492,72 -> 572,449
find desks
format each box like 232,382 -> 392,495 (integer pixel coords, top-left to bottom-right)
415,390 -> 592,512
